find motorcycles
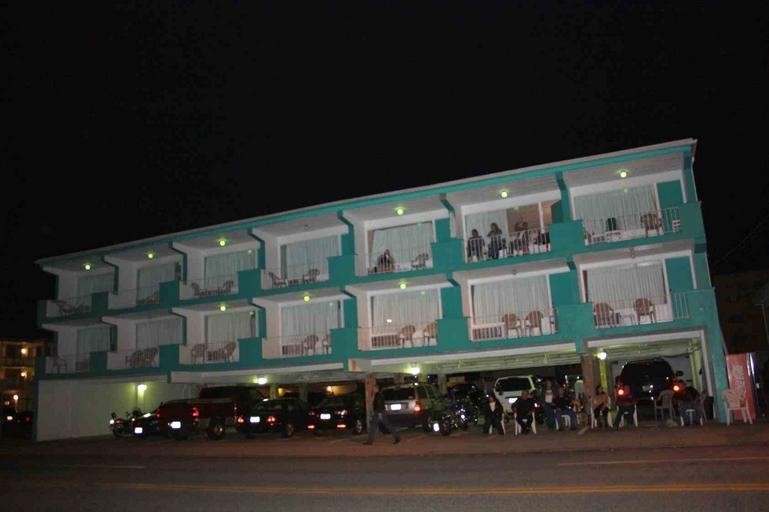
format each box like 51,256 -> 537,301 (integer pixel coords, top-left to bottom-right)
437,385 -> 479,435
110,401 -> 163,438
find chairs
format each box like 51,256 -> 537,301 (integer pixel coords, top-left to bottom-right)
134,292 -> 156,304
127,346 -> 158,366
280,332 -> 330,357
190,280 -> 236,296
370,323 -> 437,348
473,309 -> 554,338
190,340 -> 237,363
593,296 -> 658,328
581,212 -> 660,245
464,227 -> 550,261
266,267 -> 321,287
484,388 -> 754,431
366,251 -> 430,274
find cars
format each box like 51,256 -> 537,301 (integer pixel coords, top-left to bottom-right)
307,392 -> 366,436
236,398 -> 310,438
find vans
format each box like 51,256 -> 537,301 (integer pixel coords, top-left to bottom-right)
380,382 -> 448,432
616,358 -> 683,406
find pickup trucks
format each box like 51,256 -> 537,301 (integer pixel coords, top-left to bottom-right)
159,386 -> 266,440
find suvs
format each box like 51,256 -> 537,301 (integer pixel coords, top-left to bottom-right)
493,374 -> 542,419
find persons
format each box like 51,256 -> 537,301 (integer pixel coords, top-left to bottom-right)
672,380 -> 704,425
506,217 -> 529,257
482,393 -> 504,434
466,229 -> 486,262
378,250 -> 393,269
591,383 -> 610,428
612,385 -> 635,430
485,222 -> 505,260
363,385 -> 402,444
509,380 -> 581,433
